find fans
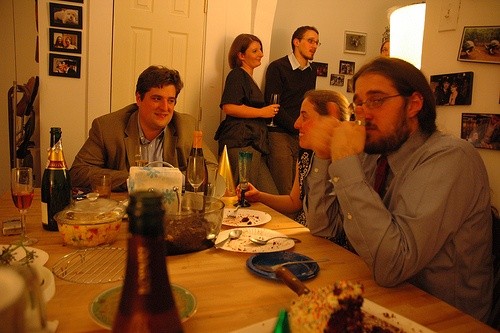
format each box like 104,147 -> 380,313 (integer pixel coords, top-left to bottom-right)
9,76 -> 39,169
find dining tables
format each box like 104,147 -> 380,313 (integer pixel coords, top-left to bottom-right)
0,192 -> 498,333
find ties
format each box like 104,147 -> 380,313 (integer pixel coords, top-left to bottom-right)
373,155 -> 388,197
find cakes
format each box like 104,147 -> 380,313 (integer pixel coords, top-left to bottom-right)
285,279 -> 365,333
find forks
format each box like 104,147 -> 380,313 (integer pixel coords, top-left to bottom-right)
228,205 -> 241,218
255,257 -> 331,272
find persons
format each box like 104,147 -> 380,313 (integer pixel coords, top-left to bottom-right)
54,36 -> 64,48
69,65 -> 218,193
467,114 -> 500,149
307,57 -> 494,325
435,79 -> 465,105
380,41 -> 390,57
213,35 -> 280,195
65,37 -> 77,49
264,26 -> 321,194
57,60 -> 75,73
237,90 -> 378,239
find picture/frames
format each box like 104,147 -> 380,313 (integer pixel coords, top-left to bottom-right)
457,26 -> 500,65
49,2 -> 82,29
49,28 -> 81,54
461,113 -> 500,151
339,60 -> 355,75
343,30 -> 368,55
430,71 -> 473,105
312,62 -> 328,77
347,79 -> 352,92
330,74 -> 344,86
49,53 -> 81,78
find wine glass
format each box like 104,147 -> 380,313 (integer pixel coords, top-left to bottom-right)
186,156 -> 206,194
11,167 -> 40,247
134,145 -> 148,167
234,151 -> 253,208
265,93 -> 280,127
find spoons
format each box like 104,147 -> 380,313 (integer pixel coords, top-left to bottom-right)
214,230 -> 243,249
249,237 -> 302,245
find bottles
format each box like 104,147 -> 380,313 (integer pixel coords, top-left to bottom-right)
110,189 -> 185,333
184,130 -> 208,195
41,127 -> 72,232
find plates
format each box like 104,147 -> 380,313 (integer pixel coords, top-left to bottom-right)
88,282 -> 199,330
0,244 -> 50,267
222,208 -> 272,227
246,251 -> 320,282
230,298 -> 437,333
214,227 -> 296,253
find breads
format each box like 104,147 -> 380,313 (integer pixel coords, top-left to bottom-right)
165,216 -> 211,254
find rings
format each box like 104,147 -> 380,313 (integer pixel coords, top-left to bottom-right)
355,120 -> 365,125
275,109 -> 276,112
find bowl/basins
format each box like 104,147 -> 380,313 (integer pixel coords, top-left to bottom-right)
119,192 -> 225,256
53,193 -> 126,250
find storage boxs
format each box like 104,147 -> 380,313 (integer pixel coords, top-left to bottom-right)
2,217 -> 22,235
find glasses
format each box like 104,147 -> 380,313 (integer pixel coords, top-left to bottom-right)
298,38 -> 321,47
348,93 -> 403,115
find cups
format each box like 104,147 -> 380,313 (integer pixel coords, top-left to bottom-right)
91,174 -> 112,200
207,162 -> 220,196
